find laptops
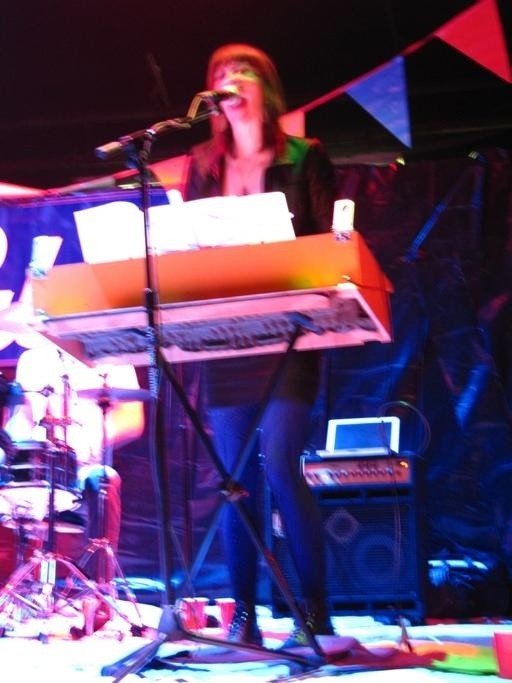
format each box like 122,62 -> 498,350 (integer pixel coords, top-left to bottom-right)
315,412 -> 402,459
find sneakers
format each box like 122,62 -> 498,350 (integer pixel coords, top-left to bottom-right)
227,602 -> 264,645
283,608 -> 335,648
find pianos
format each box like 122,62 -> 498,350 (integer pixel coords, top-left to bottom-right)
31,230 -> 394,366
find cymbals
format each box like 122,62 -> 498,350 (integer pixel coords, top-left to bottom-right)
76,390 -> 152,401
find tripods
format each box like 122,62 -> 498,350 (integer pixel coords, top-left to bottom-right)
96,105 -> 311,682
0,408 -> 147,638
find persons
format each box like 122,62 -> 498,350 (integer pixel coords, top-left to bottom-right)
178,44 -> 344,653
0,340 -> 145,634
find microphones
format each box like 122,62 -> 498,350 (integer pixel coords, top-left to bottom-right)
205,84 -> 240,103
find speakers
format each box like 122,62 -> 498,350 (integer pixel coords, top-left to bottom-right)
314,483 -> 420,618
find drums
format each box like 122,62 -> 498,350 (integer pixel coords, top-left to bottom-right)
0,441 -> 82,518
